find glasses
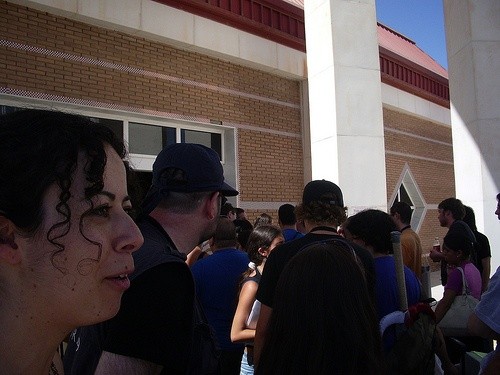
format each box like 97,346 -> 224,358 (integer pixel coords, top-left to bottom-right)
298,239 -> 356,265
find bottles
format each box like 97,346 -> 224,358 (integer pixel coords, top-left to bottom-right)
432,237 -> 441,263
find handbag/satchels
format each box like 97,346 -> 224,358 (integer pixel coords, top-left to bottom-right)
438,266 -> 481,338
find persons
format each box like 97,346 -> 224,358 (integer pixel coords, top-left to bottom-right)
65,110 -> 500,375
0,109 -> 144,375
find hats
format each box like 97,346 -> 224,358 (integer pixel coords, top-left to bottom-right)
151,143 -> 238,196
302,179 -> 344,208
